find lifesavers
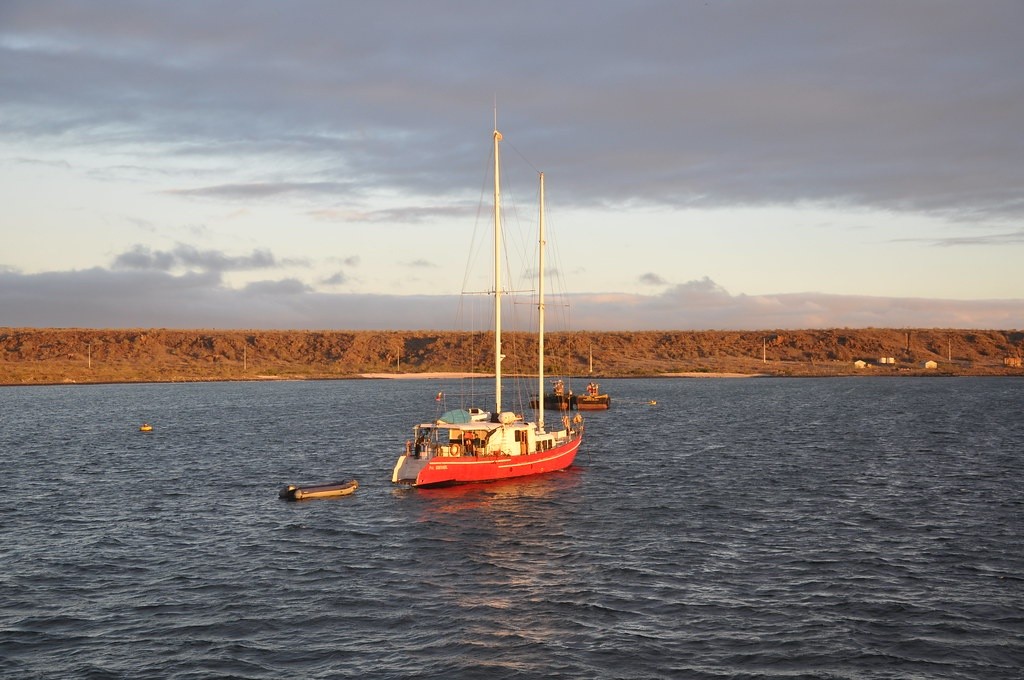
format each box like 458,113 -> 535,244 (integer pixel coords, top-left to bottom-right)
563,416 -> 568,428
450,444 -> 460,456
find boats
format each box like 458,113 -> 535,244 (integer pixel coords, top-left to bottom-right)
278,479 -> 359,500
140,426 -> 154,431
529,391 -> 610,410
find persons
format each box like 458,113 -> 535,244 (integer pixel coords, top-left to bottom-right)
474,434 -> 480,445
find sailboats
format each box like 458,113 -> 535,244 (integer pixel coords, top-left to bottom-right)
390,97 -> 584,489
